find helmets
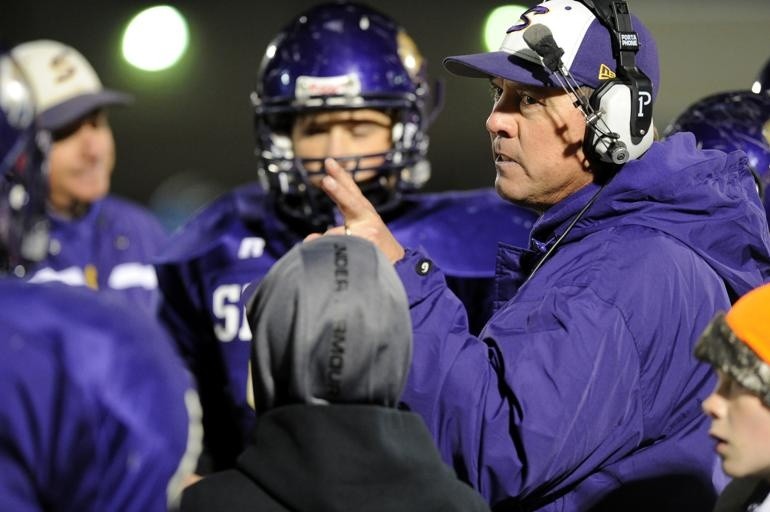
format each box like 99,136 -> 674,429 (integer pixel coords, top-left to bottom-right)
0,51 -> 52,280
663,92 -> 770,205
250,2 -> 431,225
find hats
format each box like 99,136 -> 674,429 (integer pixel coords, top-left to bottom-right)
442,1 -> 660,105
12,39 -> 135,129
692,282 -> 770,413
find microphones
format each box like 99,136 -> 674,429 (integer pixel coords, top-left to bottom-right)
522,23 -> 630,167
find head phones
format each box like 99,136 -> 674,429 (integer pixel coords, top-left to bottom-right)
582,0 -> 655,166
